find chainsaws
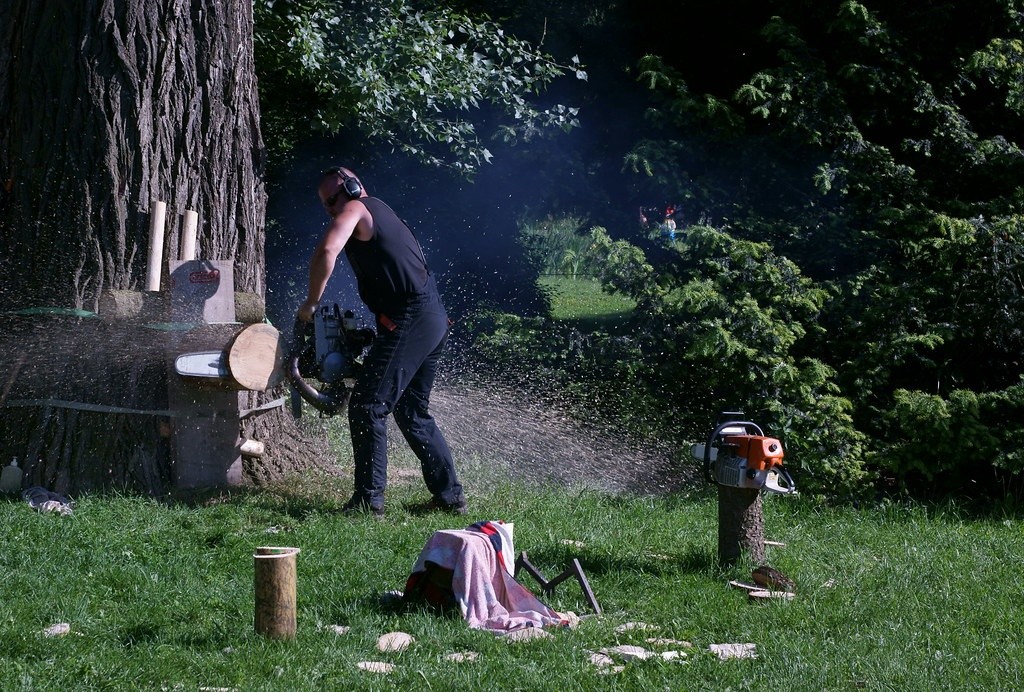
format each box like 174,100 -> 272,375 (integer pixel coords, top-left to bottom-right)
689,409 -> 797,498
172,300 -> 378,421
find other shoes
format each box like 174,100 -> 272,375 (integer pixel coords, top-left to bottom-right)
406,496 -> 468,514
331,498 -> 384,515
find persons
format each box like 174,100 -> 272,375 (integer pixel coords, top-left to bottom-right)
296,166 -> 467,514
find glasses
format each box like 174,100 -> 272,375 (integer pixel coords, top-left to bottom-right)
322,188 -> 344,208
